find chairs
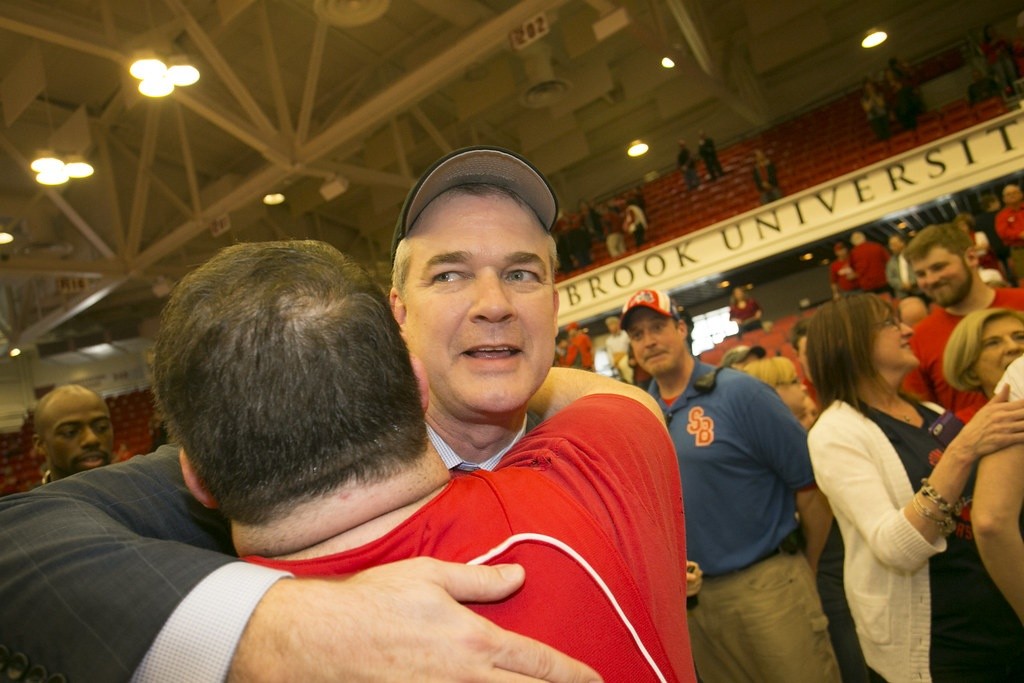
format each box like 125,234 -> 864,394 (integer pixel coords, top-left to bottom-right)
0,388 -> 162,499
554,41 -> 1008,282
699,306 -> 817,377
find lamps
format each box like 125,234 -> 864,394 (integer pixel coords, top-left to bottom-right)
31,90 -> 95,185
128,0 -> 201,98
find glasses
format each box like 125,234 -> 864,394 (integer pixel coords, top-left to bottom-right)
876,314 -> 899,328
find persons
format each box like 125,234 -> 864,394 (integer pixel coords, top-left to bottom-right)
155,236 -> 698,683
897,295 -> 929,327
938,307 -> 1024,425
955,182 -> 1024,288
978,23 -> 1020,95
33,384 -> 113,492
897,222 -> 1024,424
970,353 -> 1024,627
803,293 -> 1024,683
829,241 -> 861,299
619,287 -> 843,683
728,287 -> 763,332
554,315 -> 655,394
884,233 -> 933,306
0,143 -> 605,682
550,188 -> 647,272
750,150 -> 783,203
861,57 -> 922,140
849,231 -> 895,297
695,131 -> 724,180
722,319 -> 889,683
675,139 -> 700,189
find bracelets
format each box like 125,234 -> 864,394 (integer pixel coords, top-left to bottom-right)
913,493 -> 959,539
922,480 -> 956,515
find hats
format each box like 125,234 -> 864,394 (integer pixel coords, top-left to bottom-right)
618,290 -> 680,330
391,145 -> 559,266
